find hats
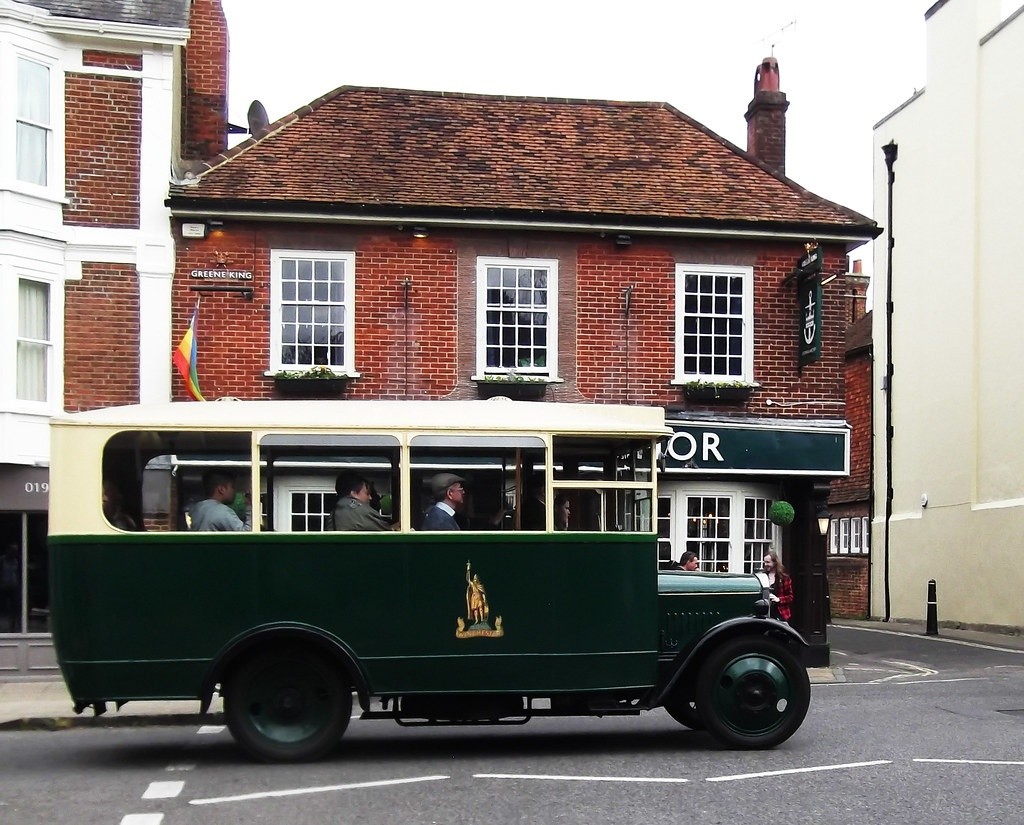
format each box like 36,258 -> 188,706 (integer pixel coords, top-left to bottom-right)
431,473 -> 466,494
335,470 -> 364,491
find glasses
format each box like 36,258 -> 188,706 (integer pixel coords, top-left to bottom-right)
456,487 -> 464,491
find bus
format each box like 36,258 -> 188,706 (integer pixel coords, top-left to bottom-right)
46,395 -> 812,764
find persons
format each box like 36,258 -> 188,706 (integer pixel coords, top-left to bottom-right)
673,551 -> 700,571
763,553 -> 794,619
103,467 -> 570,532
0,545 -> 19,615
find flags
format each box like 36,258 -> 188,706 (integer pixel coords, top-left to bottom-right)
171,296 -> 206,403
